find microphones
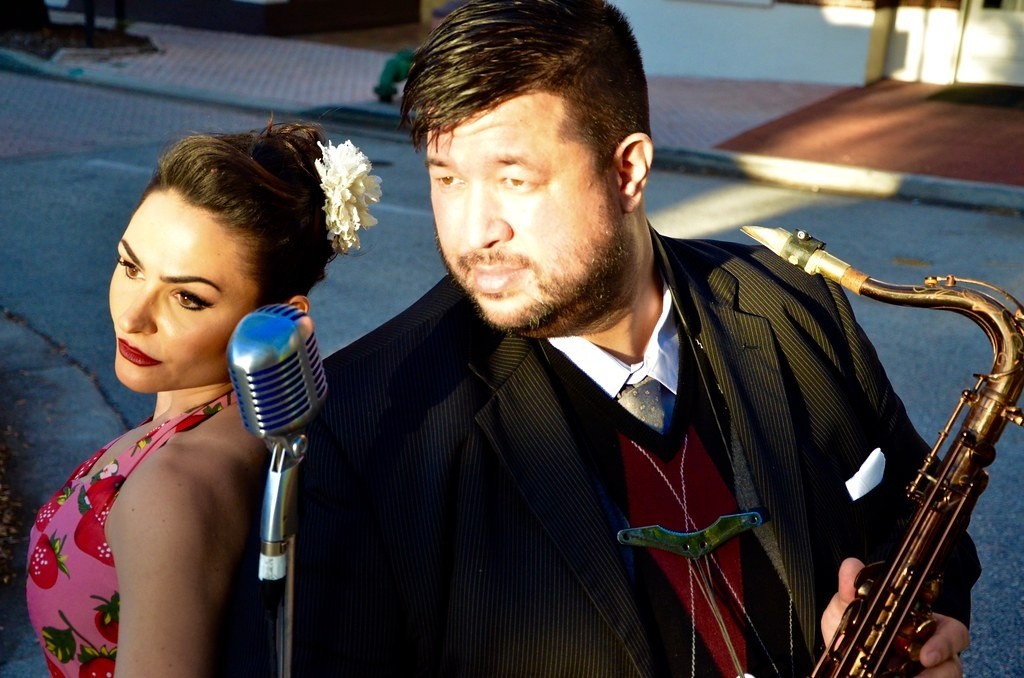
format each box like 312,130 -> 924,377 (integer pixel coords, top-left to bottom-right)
225,302 -> 329,614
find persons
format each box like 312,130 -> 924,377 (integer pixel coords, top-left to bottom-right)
23,119 -> 339,678
248,0 -> 983,678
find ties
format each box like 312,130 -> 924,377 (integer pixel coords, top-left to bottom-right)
619,379 -> 683,436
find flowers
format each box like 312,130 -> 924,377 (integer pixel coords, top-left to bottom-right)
317,141 -> 382,253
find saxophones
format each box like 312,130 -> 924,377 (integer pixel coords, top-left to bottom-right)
735,221 -> 1024,678
